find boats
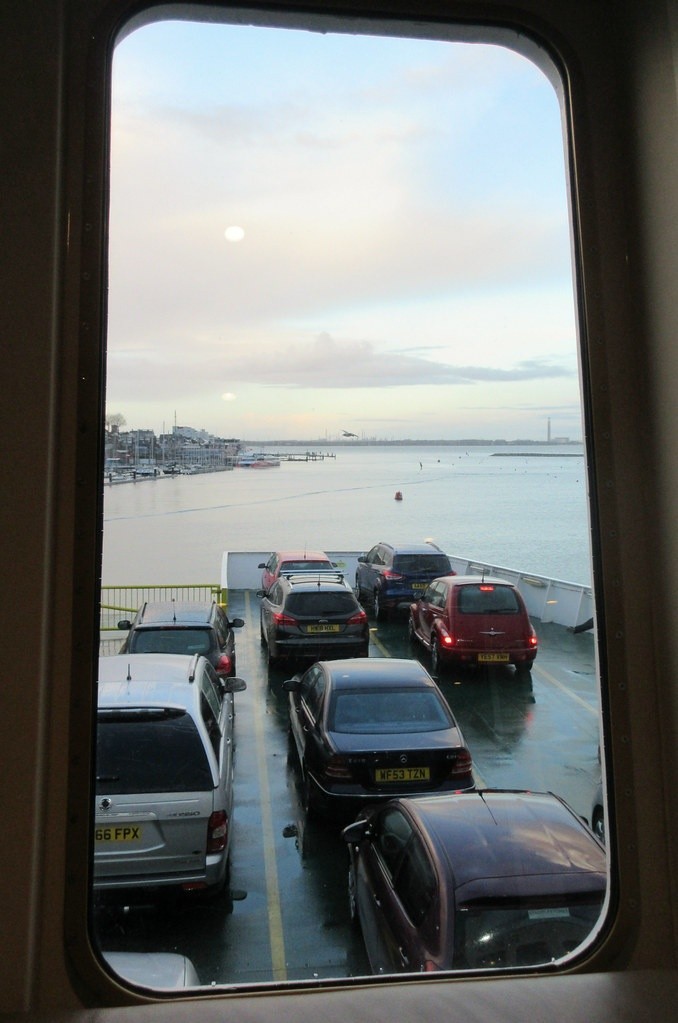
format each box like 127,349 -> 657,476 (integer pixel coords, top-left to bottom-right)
237,455 -> 282,470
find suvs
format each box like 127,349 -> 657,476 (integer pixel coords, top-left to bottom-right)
254,573 -> 372,663
354,539 -> 463,616
97,652 -> 256,929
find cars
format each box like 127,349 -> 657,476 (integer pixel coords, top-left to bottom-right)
115,600 -> 246,685
286,656 -> 475,820
342,787 -> 613,974
410,575 -> 545,670
256,549 -> 340,595
